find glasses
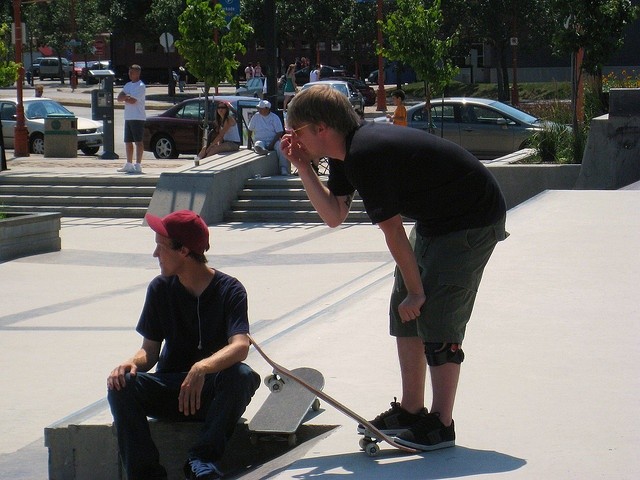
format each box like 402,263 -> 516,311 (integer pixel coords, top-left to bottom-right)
290,124 -> 312,136
218,106 -> 227,109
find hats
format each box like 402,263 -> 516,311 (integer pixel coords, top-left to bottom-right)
256,100 -> 271,109
144,210 -> 210,255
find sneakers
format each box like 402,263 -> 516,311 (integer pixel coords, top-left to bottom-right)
392,411 -> 456,452
281,167 -> 288,175
187,460 -> 222,480
255,146 -> 268,155
127,164 -> 142,173
359,402 -> 428,435
117,165 -> 134,172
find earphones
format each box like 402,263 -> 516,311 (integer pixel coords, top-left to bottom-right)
198,341 -> 203,350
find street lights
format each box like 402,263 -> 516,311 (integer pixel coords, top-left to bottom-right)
13,0 -> 51,156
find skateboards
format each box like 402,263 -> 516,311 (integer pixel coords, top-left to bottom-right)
246,333 -> 421,457
248,368 -> 325,447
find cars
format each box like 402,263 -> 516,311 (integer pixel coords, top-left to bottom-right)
143,96 -> 289,159
294,64 -> 343,86
301,81 -> 365,114
73,62 -> 87,75
319,77 -> 376,106
0,98 -> 103,154
235,77 -> 295,107
373,97 -> 573,160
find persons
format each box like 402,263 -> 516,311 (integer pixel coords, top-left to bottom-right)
280,84 -> 511,453
247,100 -> 289,176
293,58 -> 299,71
254,62 -> 265,77
301,57 -> 307,68
282,64 -> 298,112
198,101 -> 241,159
25,69 -> 31,84
34,84 -> 44,97
385,90 -> 408,127
118,64 -> 146,174
107,209 -> 262,479
177,66 -> 188,93
245,62 -> 255,83
309,64 -> 321,83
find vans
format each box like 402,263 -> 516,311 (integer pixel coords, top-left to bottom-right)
39,58 -> 73,80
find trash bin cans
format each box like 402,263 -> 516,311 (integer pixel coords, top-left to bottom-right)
45,114 -> 78,158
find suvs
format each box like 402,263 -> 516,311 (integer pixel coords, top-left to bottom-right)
30,58 -> 41,76
